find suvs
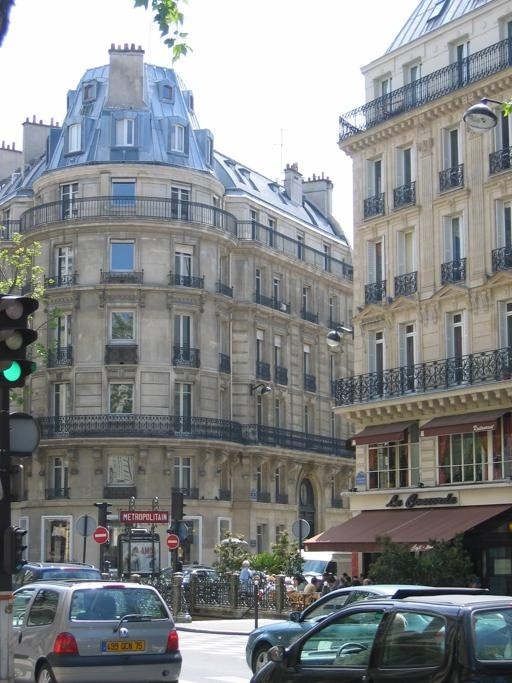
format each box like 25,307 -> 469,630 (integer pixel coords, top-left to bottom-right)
16,560 -> 100,591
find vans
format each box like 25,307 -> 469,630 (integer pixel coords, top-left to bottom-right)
11,582 -> 183,682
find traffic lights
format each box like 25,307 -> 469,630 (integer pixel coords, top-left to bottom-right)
171,492 -> 188,520
4,525 -> 29,572
0,293 -> 39,390
94,502 -> 113,525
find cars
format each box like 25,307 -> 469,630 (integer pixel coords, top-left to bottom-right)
250,595 -> 512,683
244,583 -> 509,676
156,564 -> 221,592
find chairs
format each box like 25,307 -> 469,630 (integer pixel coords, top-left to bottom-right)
288,590 -> 322,609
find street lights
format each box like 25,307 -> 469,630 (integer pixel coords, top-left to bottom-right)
150,495 -> 159,577
127,495 -> 134,571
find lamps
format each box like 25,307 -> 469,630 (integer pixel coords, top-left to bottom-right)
250,381 -> 273,397
325,324 -> 355,348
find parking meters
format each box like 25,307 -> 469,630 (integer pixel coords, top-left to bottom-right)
251,572 -> 262,629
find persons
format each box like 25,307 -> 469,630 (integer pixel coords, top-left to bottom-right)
240,559 -> 373,595
331,612 -> 408,665
111,385 -> 133,413
123,545 -> 159,569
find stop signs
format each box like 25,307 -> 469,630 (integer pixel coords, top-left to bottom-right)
92,526 -> 109,544
166,535 -> 180,549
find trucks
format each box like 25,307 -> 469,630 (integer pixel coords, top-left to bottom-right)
285,550 -> 354,587
